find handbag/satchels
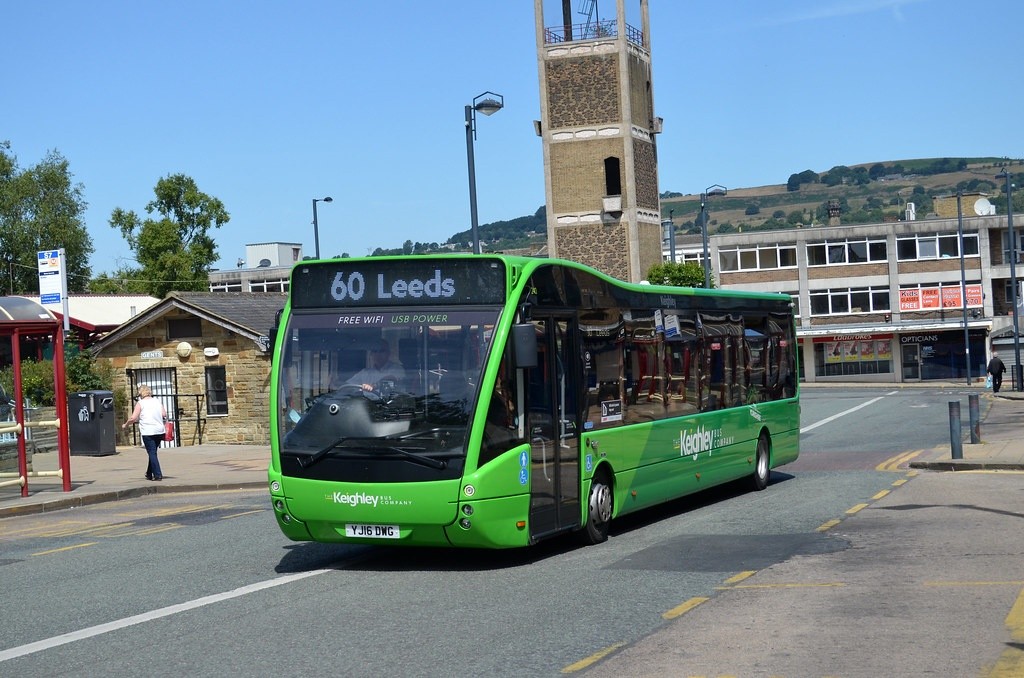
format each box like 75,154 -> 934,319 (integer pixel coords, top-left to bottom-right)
162,416 -> 175,441
987,375 -> 991,388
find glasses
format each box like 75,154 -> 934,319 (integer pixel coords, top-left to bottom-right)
371,349 -> 387,353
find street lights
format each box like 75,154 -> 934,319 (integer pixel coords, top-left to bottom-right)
956,192 -> 973,386
313,197 -> 333,260
1006,172 -> 1023,392
700,184 -> 727,289
464,91 -> 504,254
669,225 -> 679,265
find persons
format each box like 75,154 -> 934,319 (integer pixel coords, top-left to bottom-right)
327,339 -> 406,400
122,385 -> 166,482
987,352 -> 1006,393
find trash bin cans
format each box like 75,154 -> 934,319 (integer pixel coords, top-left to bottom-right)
67,390 -> 116,456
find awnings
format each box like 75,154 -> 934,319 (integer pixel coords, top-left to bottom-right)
992,337 -> 1024,344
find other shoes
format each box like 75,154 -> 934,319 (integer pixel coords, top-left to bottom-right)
152,478 -> 162,481
145,474 -> 152,480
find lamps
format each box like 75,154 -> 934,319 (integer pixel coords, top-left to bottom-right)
971,308 -> 981,319
885,315 -> 890,323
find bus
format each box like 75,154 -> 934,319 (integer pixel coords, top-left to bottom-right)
265,250 -> 804,549
